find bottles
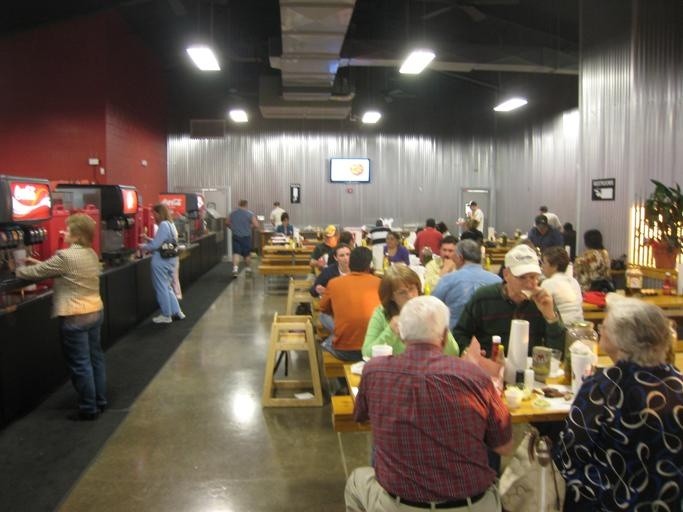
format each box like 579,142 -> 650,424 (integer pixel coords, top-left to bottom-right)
564,320 -> 599,386
288,227 -> 523,298
662,272 -> 671,295
490,333 -> 508,393
670,270 -> 678,295
514,371 -> 529,403
624,264 -> 644,296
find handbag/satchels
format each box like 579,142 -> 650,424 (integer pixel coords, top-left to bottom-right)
161,238 -> 177,257
498,422 -> 566,512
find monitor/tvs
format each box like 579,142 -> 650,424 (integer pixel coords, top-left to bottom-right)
330,157 -> 371,184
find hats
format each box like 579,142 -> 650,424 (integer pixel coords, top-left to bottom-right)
504,244 -> 542,277
467,200 -> 476,206
535,215 -> 547,225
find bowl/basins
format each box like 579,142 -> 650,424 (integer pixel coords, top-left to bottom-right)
503,388 -> 526,407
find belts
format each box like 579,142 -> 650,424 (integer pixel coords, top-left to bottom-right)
385,491 -> 484,509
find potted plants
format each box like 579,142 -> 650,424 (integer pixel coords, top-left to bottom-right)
636,175 -> 682,269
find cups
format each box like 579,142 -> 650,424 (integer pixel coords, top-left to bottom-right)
531,345 -> 563,386
371,344 -> 393,357
569,349 -> 594,396
12,249 -> 27,267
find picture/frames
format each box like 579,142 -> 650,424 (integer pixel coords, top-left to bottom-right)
590,178 -> 616,201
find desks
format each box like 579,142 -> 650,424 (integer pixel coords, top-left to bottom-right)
257,232 -> 682,433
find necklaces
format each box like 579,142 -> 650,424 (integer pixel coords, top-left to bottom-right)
275,211 -> 292,237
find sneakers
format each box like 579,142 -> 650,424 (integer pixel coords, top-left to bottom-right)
152,311 -> 186,323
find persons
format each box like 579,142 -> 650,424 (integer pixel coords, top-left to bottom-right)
550,292 -> 682,512
6,211 -> 109,421
362,265 -> 459,359
541,246 -> 584,324
309,218 -> 485,333
529,206 -> 576,260
321,246 -> 385,363
269,201 -> 286,234
224,199 -> 259,277
343,296 -> 514,512
453,244 -> 571,361
466,200 -> 484,234
136,202 -> 185,324
433,238 -> 505,331
169,222 -> 182,300
574,230 -> 611,288
